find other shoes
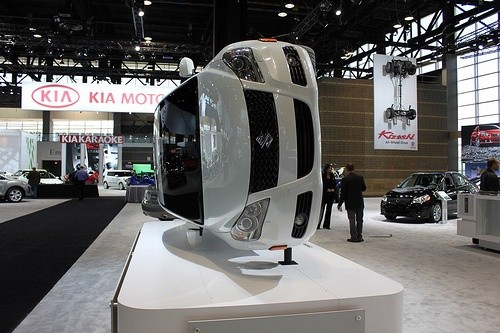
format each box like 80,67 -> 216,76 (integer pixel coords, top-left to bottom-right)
347,238 -> 364,242
323,225 -> 331,229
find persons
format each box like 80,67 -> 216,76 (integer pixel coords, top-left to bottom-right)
470,158 -> 500,245
64,170 -> 70,183
26,167 -> 40,199
83,164 -> 89,175
67,168 -> 76,183
74,166 -> 90,201
317,163 -> 337,230
18,170 -> 29,183
130,169 -> 137,178
337,162 -> 367,243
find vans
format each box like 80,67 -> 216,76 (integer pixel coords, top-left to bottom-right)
103,170 -> 137,190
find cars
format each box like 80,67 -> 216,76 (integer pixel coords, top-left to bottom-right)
152,39 -> 323,249
127,172 -> 155,186
141,184 -> 176,221
471,125 -> 500,146
181,154 -> 198,171
469,177 -> 500,192
63,170 -> 99,184
11,169 -> 64,185
164,164 -> 187,188
0,174 -> 32,203
380,171 -> 480,224
321,167 -> 345,202
